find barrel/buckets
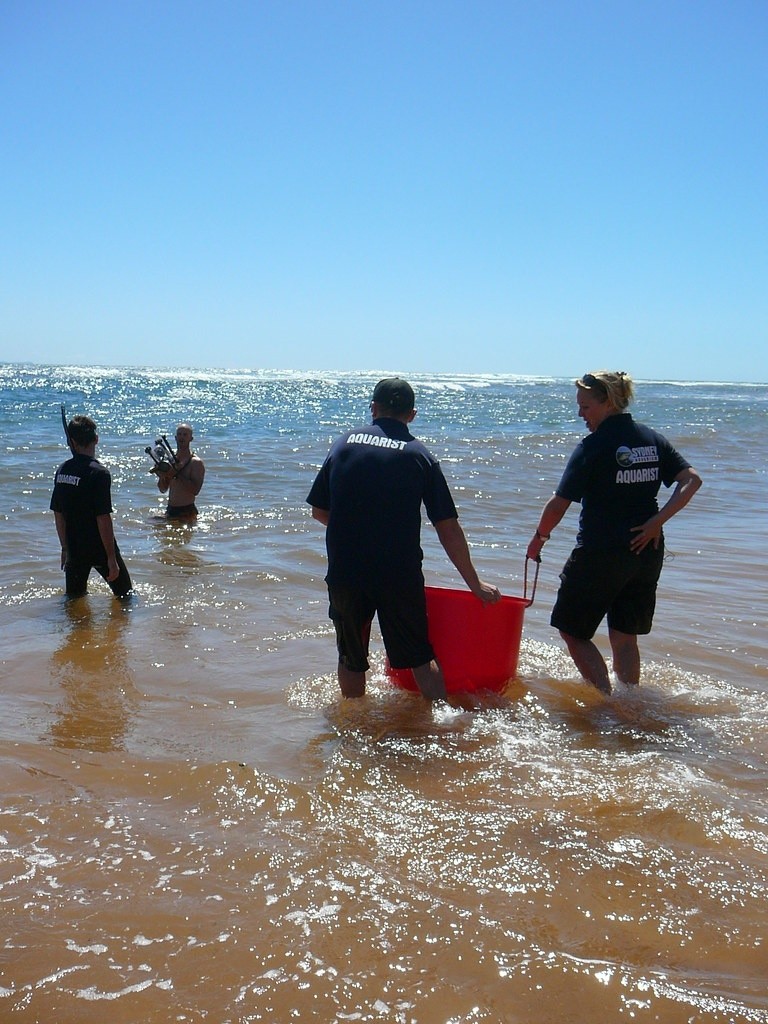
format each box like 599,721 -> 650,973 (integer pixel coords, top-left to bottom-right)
385,583 -> 533,699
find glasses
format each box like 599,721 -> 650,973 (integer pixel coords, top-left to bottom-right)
583,374 -> 608,403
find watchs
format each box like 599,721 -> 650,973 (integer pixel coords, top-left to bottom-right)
172,472 -> 178,480
537,531 -> 549,541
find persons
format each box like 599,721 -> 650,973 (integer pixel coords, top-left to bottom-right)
305,377 -> 501,703
527,371 -> 702,697
157,424 -> 204,517
50,416 -> 132,598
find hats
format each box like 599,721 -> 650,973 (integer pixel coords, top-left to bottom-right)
369,377 -> 414,411
